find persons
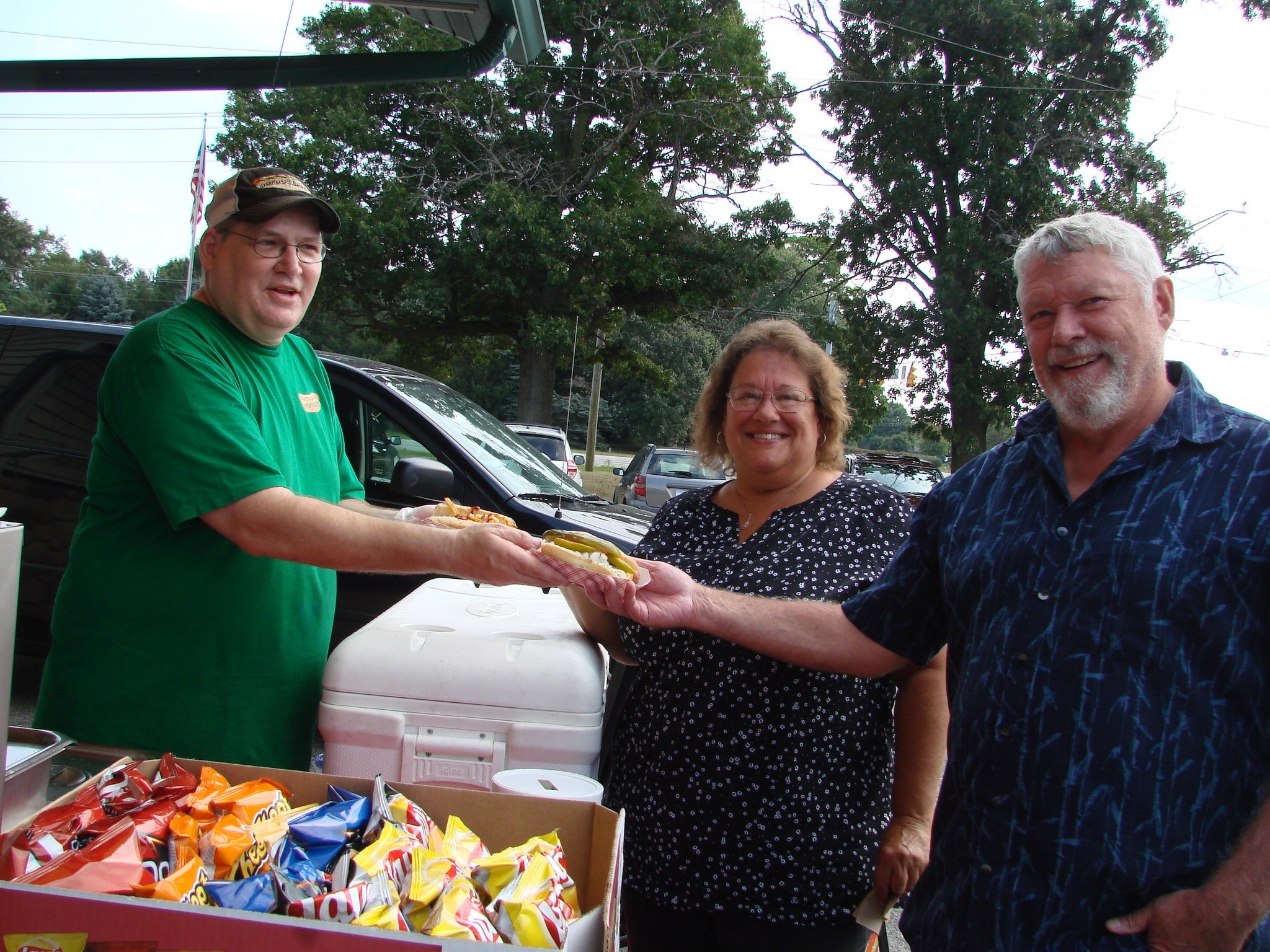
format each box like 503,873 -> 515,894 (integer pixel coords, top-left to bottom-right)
30,168 -> 569,775
558,319 -> 951,952
584,214 -> 1269,952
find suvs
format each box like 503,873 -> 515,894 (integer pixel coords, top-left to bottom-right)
612,443 -> 726,518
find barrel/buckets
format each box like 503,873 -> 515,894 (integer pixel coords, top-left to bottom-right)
491,768 -> 604,806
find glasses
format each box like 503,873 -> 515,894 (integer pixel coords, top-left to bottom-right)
214,227 -> 331,264
723,388 -> 817,411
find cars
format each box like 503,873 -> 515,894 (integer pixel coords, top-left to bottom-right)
501,420 -> 585,487
842,451 -> 944,512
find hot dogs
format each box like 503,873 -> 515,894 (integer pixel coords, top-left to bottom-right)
540,529 -> 640,585
427,498 -> 518,530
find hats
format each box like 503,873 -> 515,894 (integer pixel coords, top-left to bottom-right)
205,168 -> 341,234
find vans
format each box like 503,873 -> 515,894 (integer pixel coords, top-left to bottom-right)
0,315 -> 655,698
370,413 -> 402,480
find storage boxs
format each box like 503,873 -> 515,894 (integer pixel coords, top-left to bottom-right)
0,751 -> 625,952
320,578 -> 611,790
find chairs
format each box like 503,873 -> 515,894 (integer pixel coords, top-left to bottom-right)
688,466 -> 703,474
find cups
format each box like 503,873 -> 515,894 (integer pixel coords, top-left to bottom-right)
314,753 -> 324,773
